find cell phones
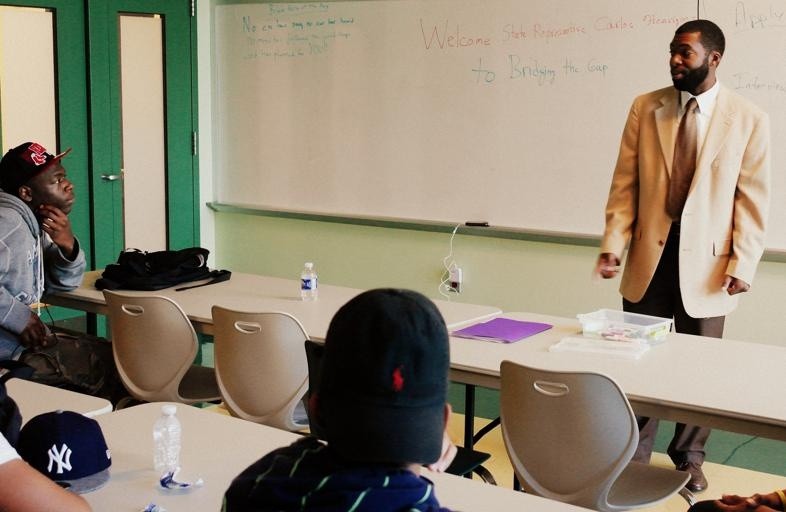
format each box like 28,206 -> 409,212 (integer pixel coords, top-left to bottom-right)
465,220 -> 489,227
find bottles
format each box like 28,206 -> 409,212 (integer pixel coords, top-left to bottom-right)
151,405 -> 181,475
299,262 -> 319,302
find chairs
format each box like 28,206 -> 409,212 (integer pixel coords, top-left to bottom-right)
212,303 -> 314,431
98,289 -> 220,405
499,358 -> 694,509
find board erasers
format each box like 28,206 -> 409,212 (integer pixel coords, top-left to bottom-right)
465,222 -> 491,226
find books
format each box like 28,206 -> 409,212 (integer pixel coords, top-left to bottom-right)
449,317 -> 555,344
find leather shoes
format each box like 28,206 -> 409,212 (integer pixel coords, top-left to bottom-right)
674,461 -> 708,492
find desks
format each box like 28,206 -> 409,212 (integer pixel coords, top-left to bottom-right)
5,369 -> 109,430
85,390 -> 592,510
450,310 -> 781,473
56,266 -> 503,359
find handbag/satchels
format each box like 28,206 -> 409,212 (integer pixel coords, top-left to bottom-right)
94,242 -> 214,292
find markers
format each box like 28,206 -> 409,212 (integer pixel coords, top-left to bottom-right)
208,0 -> 786,263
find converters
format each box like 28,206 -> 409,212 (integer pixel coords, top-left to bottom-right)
449,266 -> 462,284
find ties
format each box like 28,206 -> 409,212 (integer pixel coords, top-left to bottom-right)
664,97 -> 700,223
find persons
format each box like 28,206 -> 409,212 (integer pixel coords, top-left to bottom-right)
218,287 -> 456,511
686,489 -> 785,510
590,19 -> 775,493
0,430 -> 95,512
0,141 -> 150,412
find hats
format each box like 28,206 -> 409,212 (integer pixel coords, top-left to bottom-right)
16,409 -> 113,495
0,141 -> 73,193
303,289 -> 454,468
